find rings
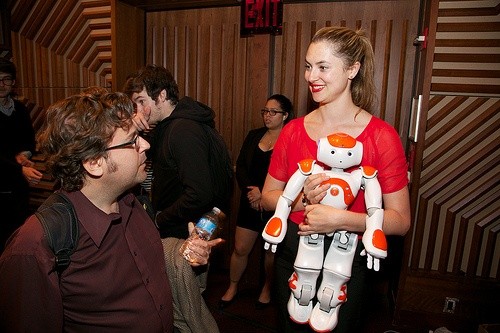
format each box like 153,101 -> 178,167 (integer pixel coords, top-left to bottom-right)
303,218 -> 308,224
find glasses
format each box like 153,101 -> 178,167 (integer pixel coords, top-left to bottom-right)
103,134 -> 143,151
260,107 -> 286,117
0,75 -> 14,87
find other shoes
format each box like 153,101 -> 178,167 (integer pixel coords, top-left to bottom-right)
256,298 -> 270,308
217,287 -> 239,308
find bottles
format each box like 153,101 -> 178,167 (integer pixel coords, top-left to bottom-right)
178,207 -> 221,262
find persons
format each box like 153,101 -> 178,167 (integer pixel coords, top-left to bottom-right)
262,132 -> 387,333
0,87 -> 222,332
0,57 -> 42,257
123,65 -> 234,276
220,94 -> 295,310
260,26 -> 410,333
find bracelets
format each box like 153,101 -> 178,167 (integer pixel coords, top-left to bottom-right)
302,194 -> 307,207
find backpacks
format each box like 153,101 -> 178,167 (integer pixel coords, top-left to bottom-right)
204,128 -> 234,216
